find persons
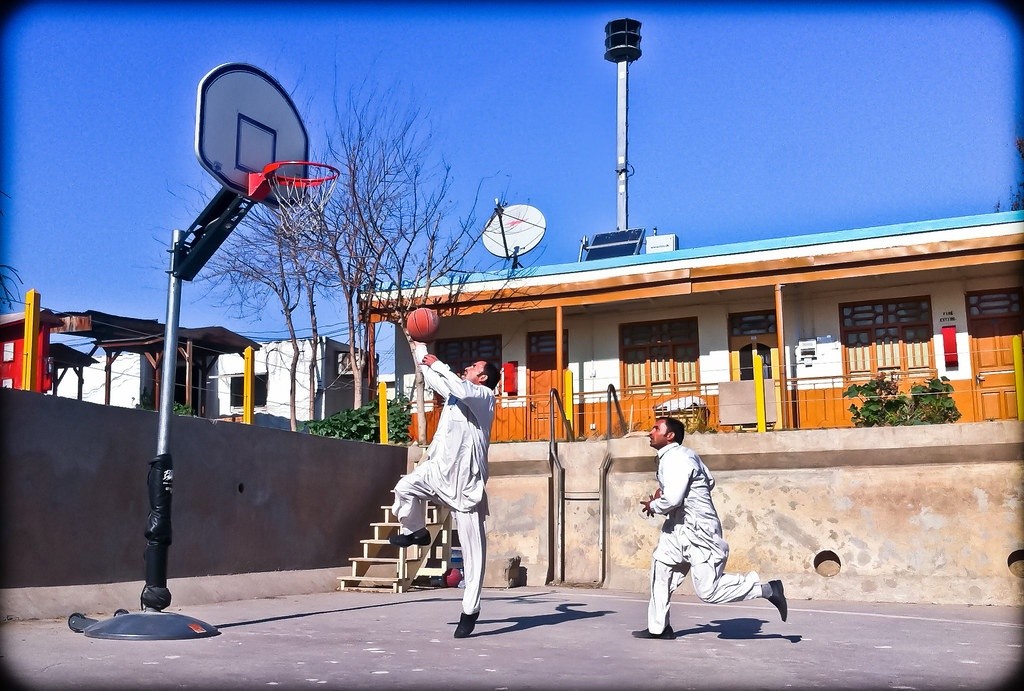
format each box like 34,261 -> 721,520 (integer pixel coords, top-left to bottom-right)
632,417 -> 788,640
388,331 -> 501,637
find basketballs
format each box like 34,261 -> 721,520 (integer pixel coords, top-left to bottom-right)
407,308 -> 438,341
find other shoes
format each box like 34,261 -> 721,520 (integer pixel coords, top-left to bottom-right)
769,580 -> 788,622
389,531 -> 431,548
632,625 -> 674,640
454,612 -> 480,638
